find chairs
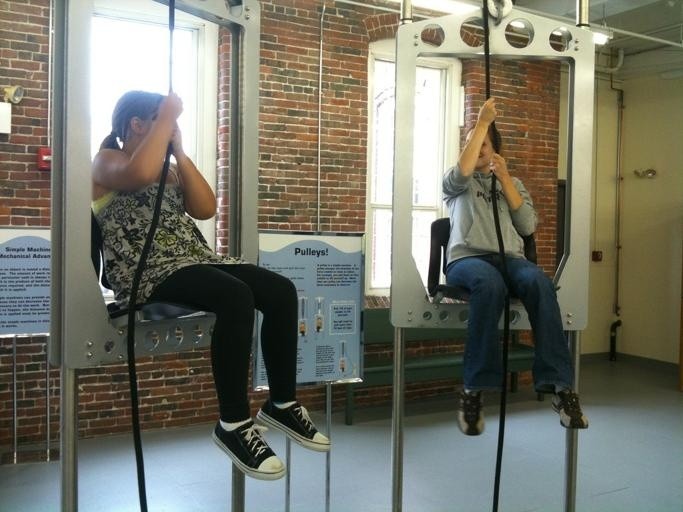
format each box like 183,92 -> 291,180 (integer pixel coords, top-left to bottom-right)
426,217 -> 538,302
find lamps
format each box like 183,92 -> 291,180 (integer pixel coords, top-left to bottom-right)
0,84 -> 25,133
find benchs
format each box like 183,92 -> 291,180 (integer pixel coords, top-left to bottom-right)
344,307 -> 535,425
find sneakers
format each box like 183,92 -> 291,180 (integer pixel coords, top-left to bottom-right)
212,421 -> 287,481
256,400 -> 331,452
456,388 -> 485,436
551,390 -> 588,428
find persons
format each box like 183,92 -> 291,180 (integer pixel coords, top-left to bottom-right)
440,96 -> 589,436
91,91 -> 331,480
